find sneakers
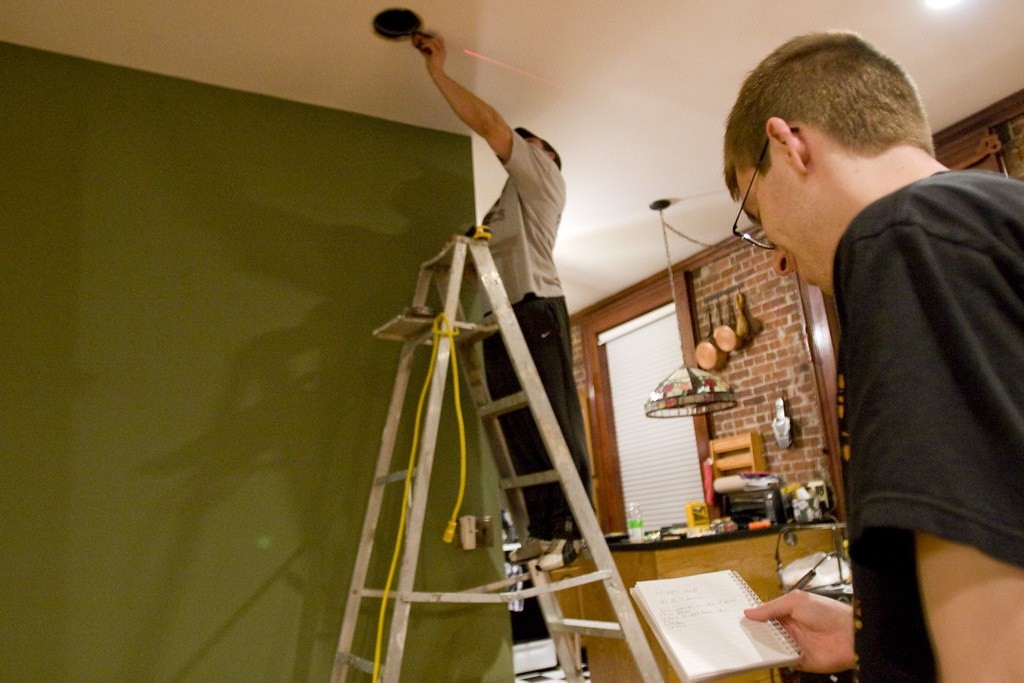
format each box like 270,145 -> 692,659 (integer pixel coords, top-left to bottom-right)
535,538 -> 585,572
508,536 -> 556,562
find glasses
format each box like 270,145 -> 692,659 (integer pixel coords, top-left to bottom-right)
733,127 -> 799,249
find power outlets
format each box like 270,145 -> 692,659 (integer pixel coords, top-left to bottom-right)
476,514 -> 495,549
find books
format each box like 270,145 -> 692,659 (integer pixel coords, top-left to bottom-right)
630,568 -> 806,683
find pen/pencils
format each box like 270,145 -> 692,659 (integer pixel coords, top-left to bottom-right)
786,570 -> 816,594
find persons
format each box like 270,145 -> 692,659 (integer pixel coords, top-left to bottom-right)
725,34 -> 1023,683
414,33 -> 595,570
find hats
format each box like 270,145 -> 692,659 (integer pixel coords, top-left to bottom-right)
514,128 -> 560,171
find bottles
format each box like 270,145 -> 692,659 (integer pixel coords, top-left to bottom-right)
627,501 -> 645,544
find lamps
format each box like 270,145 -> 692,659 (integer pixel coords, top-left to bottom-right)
644,198 -> 738,419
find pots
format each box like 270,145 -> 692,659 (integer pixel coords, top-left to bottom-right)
694,297 -> 744,371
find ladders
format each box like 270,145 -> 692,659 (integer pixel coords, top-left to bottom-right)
329,234 -> 670,683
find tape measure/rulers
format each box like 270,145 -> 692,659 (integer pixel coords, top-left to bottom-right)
465,225 -> 492,240
